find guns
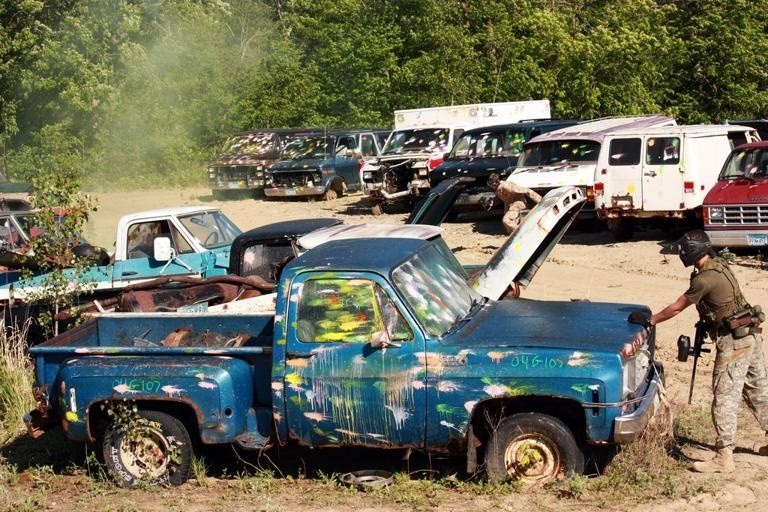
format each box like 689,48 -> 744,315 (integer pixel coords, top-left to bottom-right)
678,320 -> 709,405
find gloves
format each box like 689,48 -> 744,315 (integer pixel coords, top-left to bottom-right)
628,311 -> 654,328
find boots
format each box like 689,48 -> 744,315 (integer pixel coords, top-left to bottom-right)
693,447 -> 735,474
753,432 -> 768,456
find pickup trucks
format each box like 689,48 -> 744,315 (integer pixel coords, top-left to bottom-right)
208,184 -> 587,318
0,206 -> 244,301
55,219 -> 346,332
30,238 -> 665,490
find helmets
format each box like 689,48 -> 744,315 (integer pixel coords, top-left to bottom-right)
669,230 -> 713,267
486,173 -> 501,193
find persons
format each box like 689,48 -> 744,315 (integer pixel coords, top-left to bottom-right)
628,229 -> 768,475
486,172 -> 542,234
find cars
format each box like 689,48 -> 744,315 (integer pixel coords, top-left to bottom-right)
0,172 -> 33,195
1,198 -> 73,244
1,211 -> 115,285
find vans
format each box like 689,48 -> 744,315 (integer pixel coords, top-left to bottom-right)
264,130 -> 390,198
503,115 -> 677,235
591,120 -> 763,241
207,127 -> 343,199
428,117 -> 578,222
702,141 -> 768,248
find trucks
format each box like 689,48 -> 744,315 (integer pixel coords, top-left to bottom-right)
359,99 -> 551,214
